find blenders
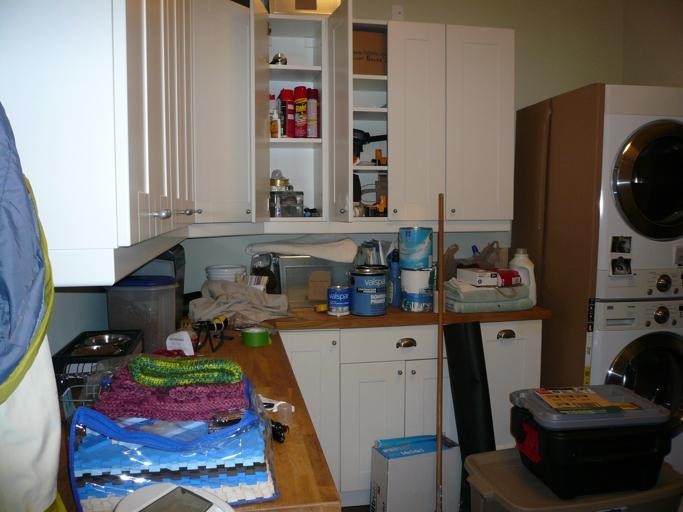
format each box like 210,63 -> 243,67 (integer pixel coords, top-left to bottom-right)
250,251 -> 277,293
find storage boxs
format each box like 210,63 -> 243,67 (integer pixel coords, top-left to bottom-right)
107,277 -> 180,354
464,449 -> 683,512
508,384 -> 671,500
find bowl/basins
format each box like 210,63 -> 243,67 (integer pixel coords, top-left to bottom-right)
207,264 -> 246,284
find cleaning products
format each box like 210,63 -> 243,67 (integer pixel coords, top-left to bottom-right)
509,248 -> 537,306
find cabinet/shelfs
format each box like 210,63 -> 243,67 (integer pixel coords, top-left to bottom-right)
339,319 -> 542,508
327,0 -> 444,234
191,0 -> 249,239
277,329 -> 340,494
445,24 -> 516,234
248,0 -> 327,234
113,1 -> 190,281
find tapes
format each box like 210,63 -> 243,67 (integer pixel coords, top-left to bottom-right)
240,327 -> 272,347
